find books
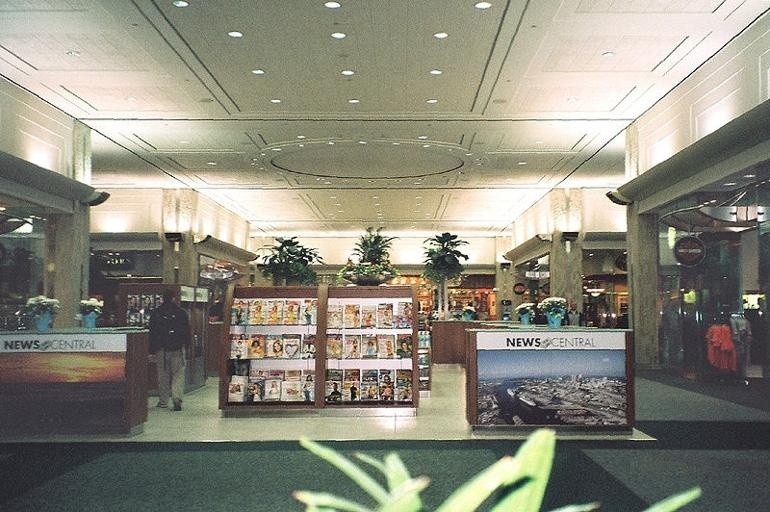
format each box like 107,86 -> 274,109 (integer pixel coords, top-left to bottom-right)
231,294 -> 413,404
126,293 -> 165,327
418,331 -> 431,390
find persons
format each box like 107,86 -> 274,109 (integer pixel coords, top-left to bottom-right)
147,284 -> 192,410
753,298 -> 766,363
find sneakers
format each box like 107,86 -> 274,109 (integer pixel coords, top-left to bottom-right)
156,397 -> 183,412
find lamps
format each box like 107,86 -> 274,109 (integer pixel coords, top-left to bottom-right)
585,280 -> 606,296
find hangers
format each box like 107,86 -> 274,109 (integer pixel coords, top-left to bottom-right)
712,313 -> 749,327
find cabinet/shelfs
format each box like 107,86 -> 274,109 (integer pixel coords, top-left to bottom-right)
0,283 -> 635,437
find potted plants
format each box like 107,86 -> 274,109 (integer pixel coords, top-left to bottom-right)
257,225 -> 401,286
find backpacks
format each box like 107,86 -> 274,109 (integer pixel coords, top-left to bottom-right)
159,307 -> 185,351
